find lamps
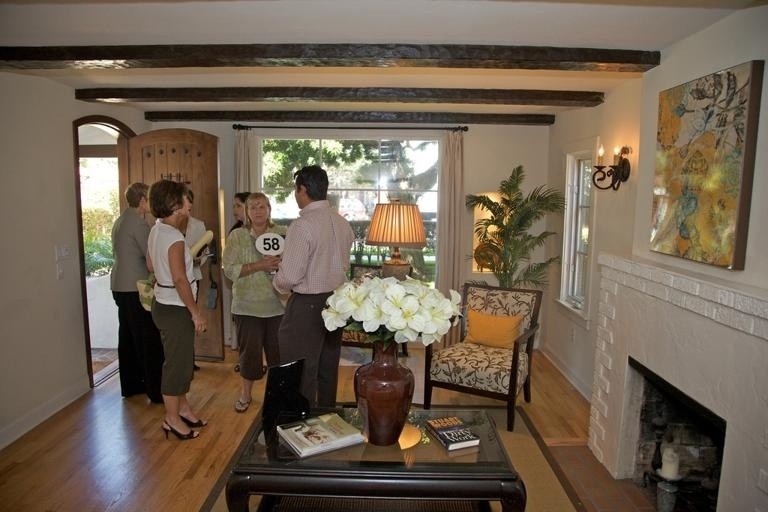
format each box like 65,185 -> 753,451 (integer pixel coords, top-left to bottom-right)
365,204 -> 428,283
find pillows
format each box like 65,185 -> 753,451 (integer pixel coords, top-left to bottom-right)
466,306 -> 521,351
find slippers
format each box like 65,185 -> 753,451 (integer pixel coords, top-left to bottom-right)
235,397 -> 252,413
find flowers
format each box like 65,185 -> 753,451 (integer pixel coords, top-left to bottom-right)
323,274 -> 464,342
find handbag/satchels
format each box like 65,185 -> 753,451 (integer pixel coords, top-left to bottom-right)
136,270 -> 157,312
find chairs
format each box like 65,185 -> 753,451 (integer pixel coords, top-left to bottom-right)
425,282 -> 544,431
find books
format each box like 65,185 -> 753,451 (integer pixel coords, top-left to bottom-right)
276,412 -> 365,459
424,416 -> 481,453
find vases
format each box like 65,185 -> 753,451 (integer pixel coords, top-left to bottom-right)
347,342 -> 415,447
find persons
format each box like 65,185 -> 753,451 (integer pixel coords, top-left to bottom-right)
272,165 -> 355,409
145,180 -> 208,440
180,189 -> 208,370
110,182 -> 166,404
229,191 -> 251,372
222,193 -> 291,414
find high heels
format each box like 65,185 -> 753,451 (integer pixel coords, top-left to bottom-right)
178,415 -> 208,427
162,420 -> 199,439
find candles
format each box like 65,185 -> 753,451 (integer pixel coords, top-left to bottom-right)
596,145 -> 605,166
612,145 -> 620,166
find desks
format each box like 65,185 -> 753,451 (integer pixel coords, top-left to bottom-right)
224,399 -> 526,512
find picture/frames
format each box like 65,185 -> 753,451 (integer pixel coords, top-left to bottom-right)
648,56 -> 768,271
